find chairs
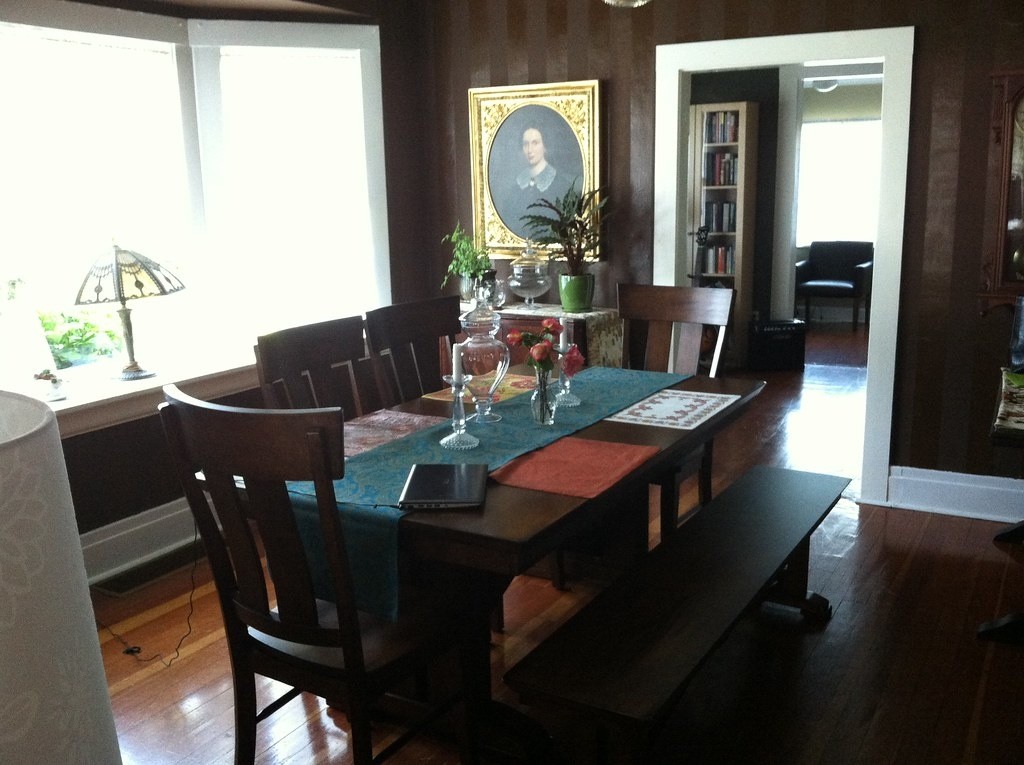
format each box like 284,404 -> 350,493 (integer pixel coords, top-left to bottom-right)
795,240 -> 874,331
255,315 -> 382,424
364,296 -> 460,407
614,282 -> 734,541
158,384 -> 462,765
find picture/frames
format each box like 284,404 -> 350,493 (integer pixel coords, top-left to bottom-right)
468,80 -> 601,261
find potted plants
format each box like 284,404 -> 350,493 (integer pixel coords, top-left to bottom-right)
440,220 -> 494,302
518,175 -> 611,313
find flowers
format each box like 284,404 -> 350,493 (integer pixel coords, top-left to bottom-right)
506,317 -> 584,421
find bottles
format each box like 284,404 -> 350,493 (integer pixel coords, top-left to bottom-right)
507,240 -> 552,310
456,285 -> 510,423
482,279 -> 505,310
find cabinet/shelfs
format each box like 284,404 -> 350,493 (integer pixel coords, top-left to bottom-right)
689,101 -> 758,361
460,302 -> 623,367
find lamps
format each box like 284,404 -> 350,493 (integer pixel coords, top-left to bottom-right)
75,248 -> 185,380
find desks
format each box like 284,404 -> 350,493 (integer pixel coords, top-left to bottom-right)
196,364 -> 765,722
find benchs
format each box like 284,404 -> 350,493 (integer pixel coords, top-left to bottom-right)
504,466 -> 852,765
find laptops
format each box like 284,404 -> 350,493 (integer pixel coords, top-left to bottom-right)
396,463 -> 488,510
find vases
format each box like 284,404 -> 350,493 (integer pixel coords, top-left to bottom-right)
531,365 -> 558,426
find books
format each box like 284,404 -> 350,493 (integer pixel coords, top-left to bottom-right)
704,246 -> 735,274
702,151 -> 739,186
706,112 -> 736,142
703,201 -> 736,233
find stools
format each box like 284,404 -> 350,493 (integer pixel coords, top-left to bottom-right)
748,319 -> 805,373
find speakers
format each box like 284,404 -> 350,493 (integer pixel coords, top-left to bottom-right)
749,318 -> 806,372
